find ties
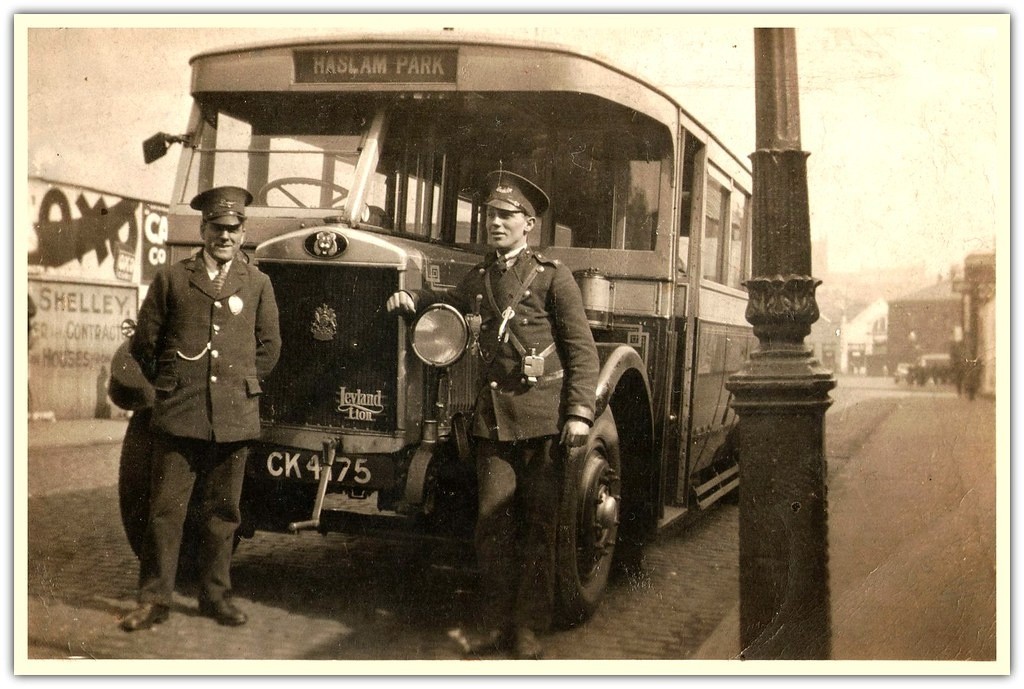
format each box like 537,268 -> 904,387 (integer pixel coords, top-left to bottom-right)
496,255 -> 507,274
212,262 -> 228,293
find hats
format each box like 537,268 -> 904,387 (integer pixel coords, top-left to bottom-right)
482,170 -> 550,217
188,186 -> 253,226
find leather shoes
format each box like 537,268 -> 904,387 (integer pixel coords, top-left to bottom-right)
122,601 -> 170,630
198,599 -> 247,626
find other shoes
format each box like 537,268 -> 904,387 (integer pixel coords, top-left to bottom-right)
508,629 -> 543,660
464,628 -> 513,652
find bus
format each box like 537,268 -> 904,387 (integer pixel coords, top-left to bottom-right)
104,27 -> 761,629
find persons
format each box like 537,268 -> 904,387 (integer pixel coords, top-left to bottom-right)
384,170 -> 600,661
904,360 -> 958,388
119,185 -> 281,634
